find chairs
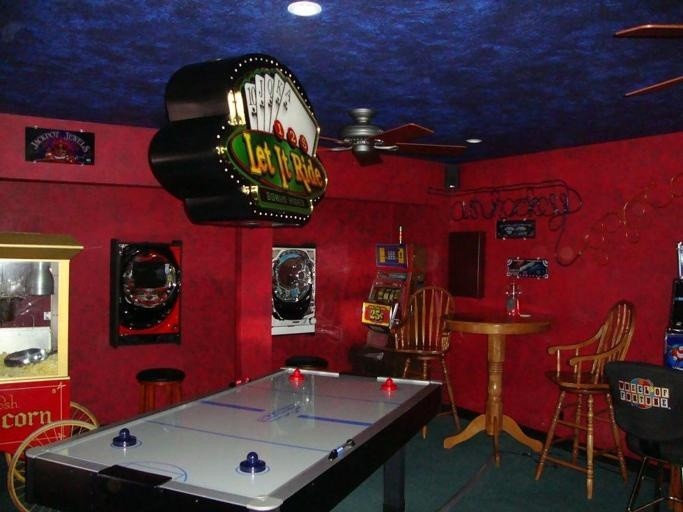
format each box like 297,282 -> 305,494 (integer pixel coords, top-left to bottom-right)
604,360 -> 683,512
535,298 -> 636,500
395,285 -> 461,439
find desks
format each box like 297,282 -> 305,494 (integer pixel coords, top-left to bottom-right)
442,314 -> 551,470
24,367 -> 443,512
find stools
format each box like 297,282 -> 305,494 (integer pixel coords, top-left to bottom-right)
285,356 -> 328,370
136,368 -> 184,414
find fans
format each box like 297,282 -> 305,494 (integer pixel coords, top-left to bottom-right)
615,24 -> 683,97
320,107 -> 468,156
